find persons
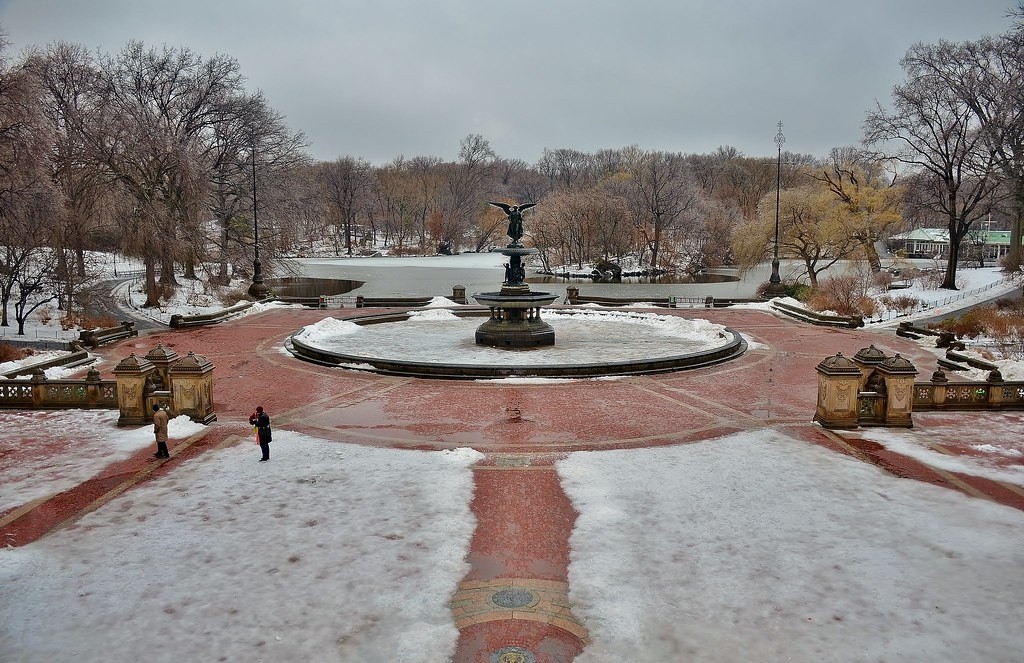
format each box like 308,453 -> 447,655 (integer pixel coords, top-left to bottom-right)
501,262 -> 512,283
516,263 -> 526,284
253,406 -> 272,462
507,205 -> 523,242
152,403 -> 170,459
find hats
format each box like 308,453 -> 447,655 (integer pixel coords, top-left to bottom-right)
256,406 -> 263,412
153,404 -> 159,412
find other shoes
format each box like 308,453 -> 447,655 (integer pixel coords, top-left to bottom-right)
260,458 -> 271,461
154,451 -> 169,457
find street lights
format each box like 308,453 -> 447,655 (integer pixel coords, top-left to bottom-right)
759,118 -> 802,300
